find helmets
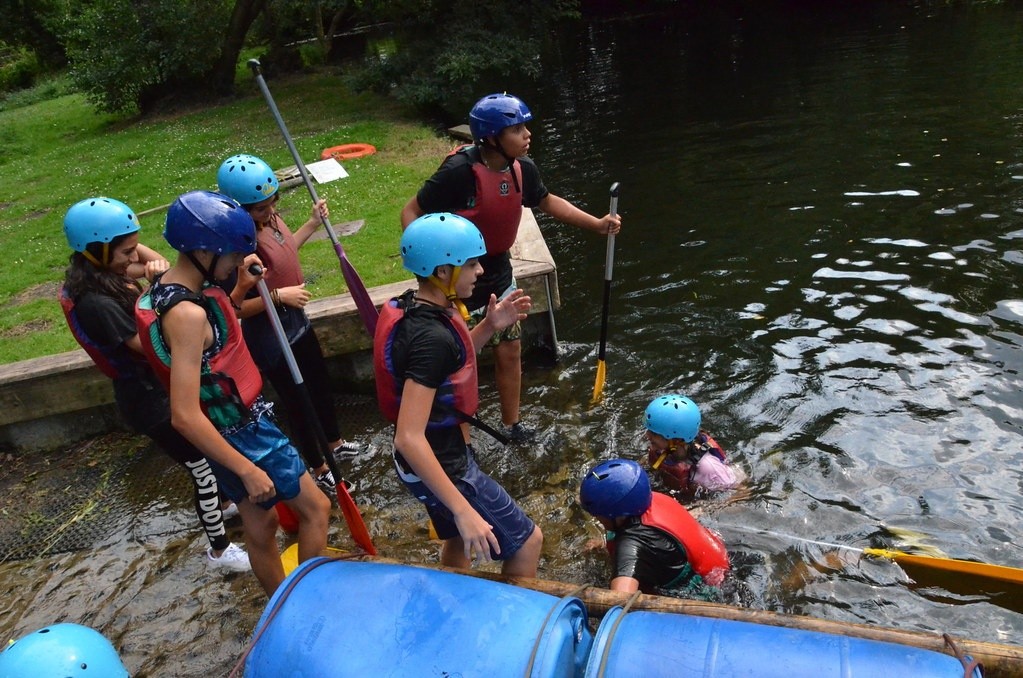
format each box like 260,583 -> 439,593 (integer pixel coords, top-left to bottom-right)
64,198 -> 141,252
164,190 -> 256,256
399,212 -> 487,278
217,154 -> 279,205
580,459 -> 651,519
468,93 -> 532,139
642,393 -> 701,443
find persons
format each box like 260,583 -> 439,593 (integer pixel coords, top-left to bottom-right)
57,196 -> 254,574
400,92 -> 621,432
374,211 -> 544,579
580,458 -> 732,594
642,394 -> 748,498
135,189 -> 331,601
217,154 -> 373,495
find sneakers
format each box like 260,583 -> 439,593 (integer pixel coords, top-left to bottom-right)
223,502 -> 239,520
206,542 -> 252,573
333,440 -> 372,456
316,469 -> 354,495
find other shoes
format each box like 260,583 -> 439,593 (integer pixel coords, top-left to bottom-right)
498,421 -> 533,444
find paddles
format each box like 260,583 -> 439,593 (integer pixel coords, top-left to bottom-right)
593,180 -> 622,404
246,54 -> 383,337
721,520 -> 1023,584
278,541 -> 349,578
248,263 -> 379,557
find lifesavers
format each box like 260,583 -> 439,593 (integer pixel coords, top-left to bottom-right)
320,143 -> 376,161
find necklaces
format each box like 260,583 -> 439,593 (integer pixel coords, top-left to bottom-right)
480,147 -> 511,174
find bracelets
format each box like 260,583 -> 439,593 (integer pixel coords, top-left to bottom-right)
228,294 -> 242,311
270,288 -> 282,307
